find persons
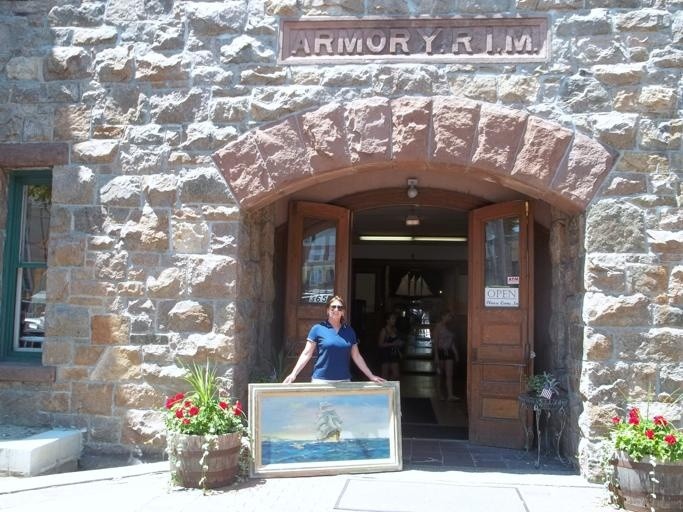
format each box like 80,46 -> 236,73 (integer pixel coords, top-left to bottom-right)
432,308 -> 462,401
377,312 -> 404,378
282,295 -> 387,385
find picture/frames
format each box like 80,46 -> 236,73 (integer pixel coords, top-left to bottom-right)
245,378 -> 405,479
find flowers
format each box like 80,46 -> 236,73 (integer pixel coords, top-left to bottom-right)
158,353 -> 254,438
607,391 -> 683,463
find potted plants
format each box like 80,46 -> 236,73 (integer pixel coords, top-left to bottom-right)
527,372 -> 561,399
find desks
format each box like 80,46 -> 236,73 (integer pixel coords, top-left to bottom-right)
517,392 -> 570,469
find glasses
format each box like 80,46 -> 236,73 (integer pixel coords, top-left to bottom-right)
329,305 -> 344,311
